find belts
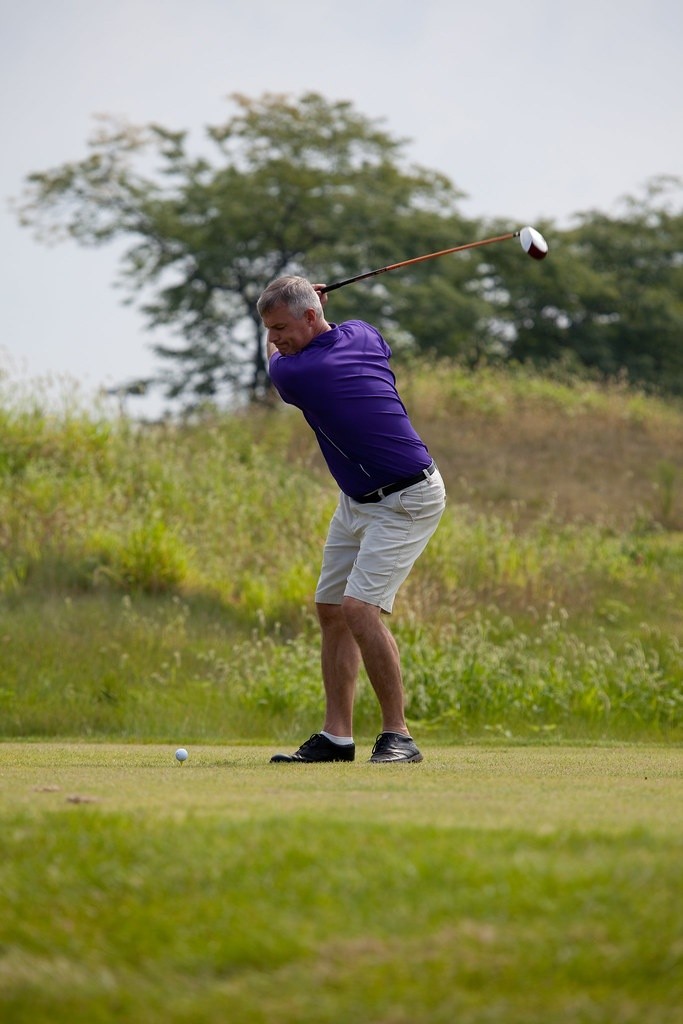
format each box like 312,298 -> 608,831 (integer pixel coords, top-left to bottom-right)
352,456 -> 435,504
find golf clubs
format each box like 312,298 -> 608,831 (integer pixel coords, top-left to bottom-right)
323,226 -> 549,292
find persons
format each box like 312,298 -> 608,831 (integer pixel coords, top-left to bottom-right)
256,274 -> 445,764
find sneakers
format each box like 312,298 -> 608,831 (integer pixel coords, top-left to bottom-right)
365,732 -> 424,767
270,734 -> 356,764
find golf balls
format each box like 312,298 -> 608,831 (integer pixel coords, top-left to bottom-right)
175,748 -> 188,761
